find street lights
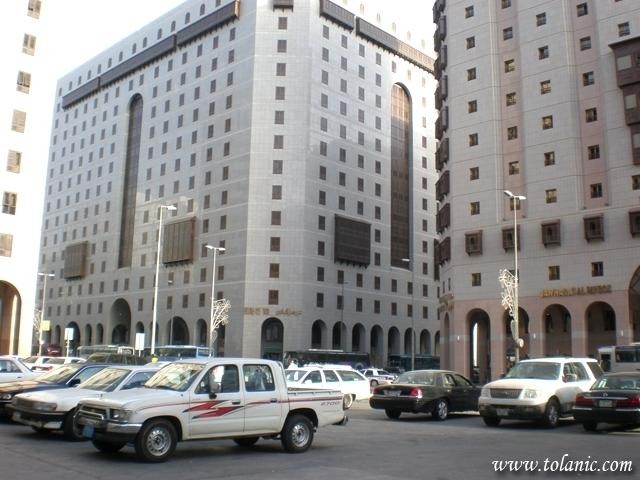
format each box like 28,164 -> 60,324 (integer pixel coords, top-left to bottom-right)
151,205 -> 177,356
37,272 -> 57,357
204,244 -> 226,358
503,189 -> 527,361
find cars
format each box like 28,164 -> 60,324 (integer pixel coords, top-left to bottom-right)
571,370 -> 640,431
368,368 -> 482,421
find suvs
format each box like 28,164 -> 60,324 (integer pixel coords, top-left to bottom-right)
478,358 -> 605,428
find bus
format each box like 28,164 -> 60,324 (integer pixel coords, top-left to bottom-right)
386,354 -> 439,374
596,343 -> 640,372
